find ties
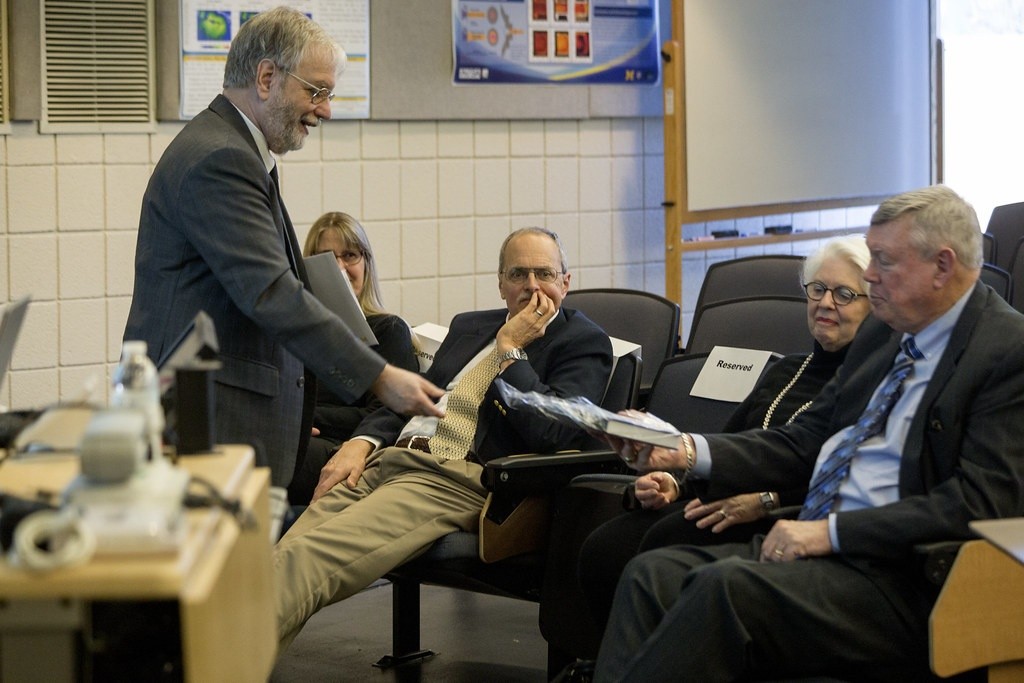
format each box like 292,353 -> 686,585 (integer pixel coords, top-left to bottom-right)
797,337 -> 923,521
428,343 -> 501,460
270,162 -> 278,190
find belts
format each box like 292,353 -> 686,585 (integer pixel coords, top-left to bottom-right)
395,435 -> 485,467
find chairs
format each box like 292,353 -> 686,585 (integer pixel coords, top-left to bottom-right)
364,204 -> 1024,683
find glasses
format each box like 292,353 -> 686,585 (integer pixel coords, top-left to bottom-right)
803,282 -> 867,306
274,62 -> 335,104
501,266 -> 563,284
322,249 -> 363,265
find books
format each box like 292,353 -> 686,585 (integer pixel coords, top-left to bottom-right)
300,252 -> 379,347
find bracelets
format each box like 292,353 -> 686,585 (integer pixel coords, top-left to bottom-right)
672,432 -> 692,485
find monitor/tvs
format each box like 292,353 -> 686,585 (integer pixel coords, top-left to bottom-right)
156,309 -> 217,417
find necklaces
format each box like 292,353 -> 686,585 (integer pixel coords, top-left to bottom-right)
762,353 -> 813,430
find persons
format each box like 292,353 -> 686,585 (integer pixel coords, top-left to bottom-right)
267,226 -> 614,679
596,183 -> 1024,683
283,212 -> 420,506
120,7 -> 448,540
580,233 -> 874,610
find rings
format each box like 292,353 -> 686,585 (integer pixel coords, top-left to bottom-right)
625,451 -> 638,462
720,509 -> 725,519
536,310 -> 543,316
775,549 -> 784,556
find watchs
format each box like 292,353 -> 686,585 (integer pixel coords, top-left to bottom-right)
759,491 -> 775,512
498,347 -> 528,368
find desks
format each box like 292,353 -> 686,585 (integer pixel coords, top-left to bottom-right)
0,441 -> 281,682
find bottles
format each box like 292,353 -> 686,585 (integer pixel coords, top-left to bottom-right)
109,340 -> 165,461
